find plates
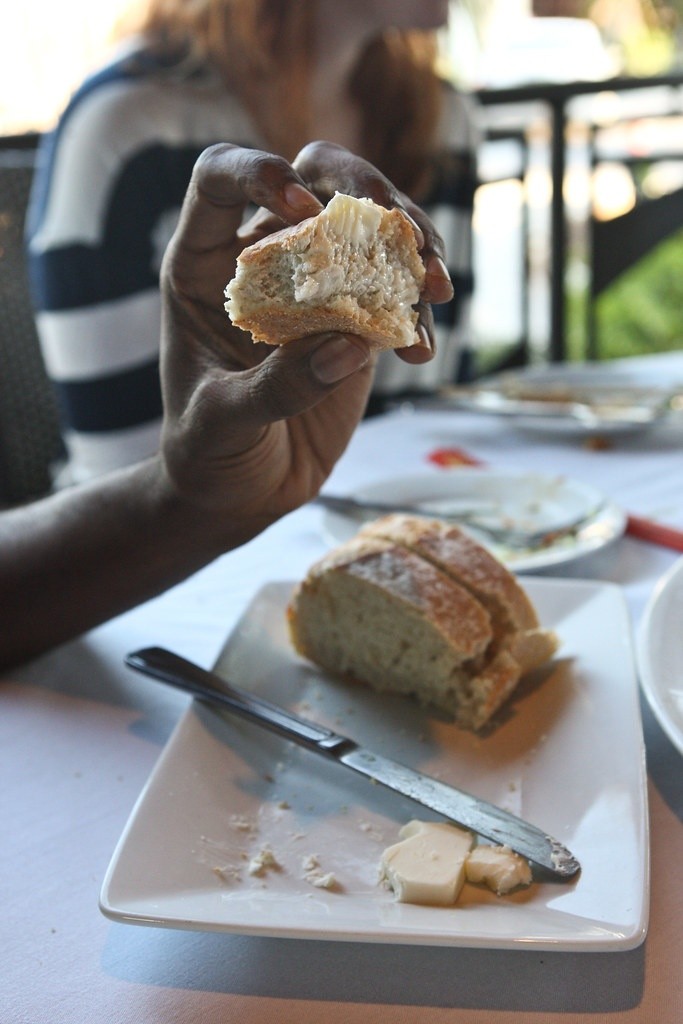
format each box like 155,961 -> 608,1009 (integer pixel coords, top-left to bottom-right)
482,376 -> 682,439
637,554 -> 683,752
100,580 -> 653,953
328,469 -> 625,575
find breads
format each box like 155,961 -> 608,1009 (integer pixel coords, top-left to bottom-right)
221,197 -> 422,347
289,511 -> 558,731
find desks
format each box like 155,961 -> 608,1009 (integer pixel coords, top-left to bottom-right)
0,349 -> 683,1024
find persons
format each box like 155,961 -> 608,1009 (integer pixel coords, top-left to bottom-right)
1,140 -> 454,687
22,0 -> 479,487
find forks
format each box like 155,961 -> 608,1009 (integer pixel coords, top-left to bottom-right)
308,495 -> 613,548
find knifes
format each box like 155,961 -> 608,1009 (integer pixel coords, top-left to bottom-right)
121,646 -> 580,878
